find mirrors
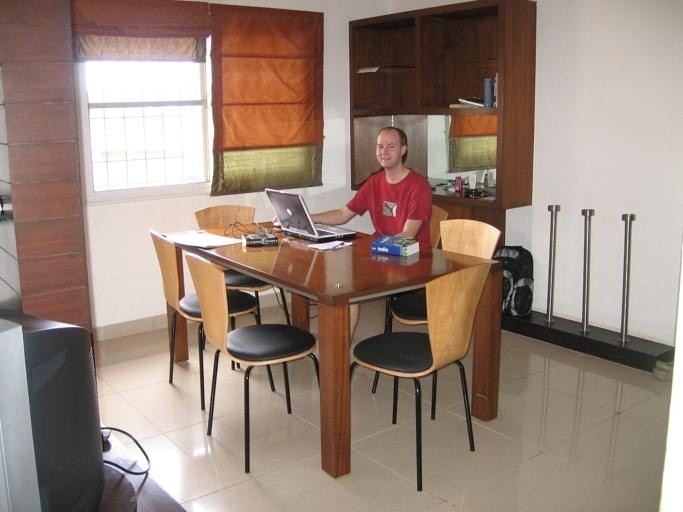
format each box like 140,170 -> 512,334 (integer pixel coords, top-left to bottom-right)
352,116 -> 497,197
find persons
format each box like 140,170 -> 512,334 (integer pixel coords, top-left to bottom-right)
272,126 -> 432,342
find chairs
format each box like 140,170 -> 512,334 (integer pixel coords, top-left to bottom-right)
194,203 -> 502,393
185,254 -> 319,473
150,231 -> 276,411
347,262 -> 493,493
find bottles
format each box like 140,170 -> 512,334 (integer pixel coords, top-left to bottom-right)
483,77 -> 494,106
454,174 -> 463,192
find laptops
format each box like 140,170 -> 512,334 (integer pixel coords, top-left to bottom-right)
265,188 -> 356,242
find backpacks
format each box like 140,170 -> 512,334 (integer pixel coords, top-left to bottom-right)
492,245 -> 533,322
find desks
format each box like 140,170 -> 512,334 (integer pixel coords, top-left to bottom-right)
163,221 -> 501,479
98,422 -> 188,512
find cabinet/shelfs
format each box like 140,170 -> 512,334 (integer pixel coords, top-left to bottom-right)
348,1 -> 536,257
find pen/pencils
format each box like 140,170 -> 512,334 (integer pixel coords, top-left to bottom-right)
333,243 -> 344,248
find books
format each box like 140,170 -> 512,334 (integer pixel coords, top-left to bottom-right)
371,235 -> 420,257
449,72 -> 499,109
